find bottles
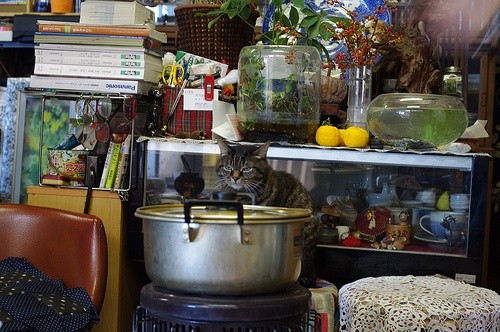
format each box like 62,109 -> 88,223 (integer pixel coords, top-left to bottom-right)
441,66 -> 464,103
381,182 -> 400,207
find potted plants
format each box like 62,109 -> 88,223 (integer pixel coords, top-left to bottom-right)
191,0 -> 345,143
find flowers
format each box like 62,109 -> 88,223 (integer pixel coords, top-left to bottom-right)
271,0 -> 402,78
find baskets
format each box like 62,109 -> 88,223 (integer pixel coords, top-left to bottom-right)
173,4 -> 260,76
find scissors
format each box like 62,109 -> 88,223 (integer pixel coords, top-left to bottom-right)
162,65 -> 184,85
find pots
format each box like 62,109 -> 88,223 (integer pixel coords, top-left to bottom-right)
134,192 -> 313,297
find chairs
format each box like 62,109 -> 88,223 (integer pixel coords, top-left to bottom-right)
0,203 -> 108,332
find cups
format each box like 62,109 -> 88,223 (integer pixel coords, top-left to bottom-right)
366,189 -> 469,240
448,216 -> 466,253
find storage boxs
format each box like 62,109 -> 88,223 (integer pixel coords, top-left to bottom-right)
143,140 -> 475,261
367,93 -> 469,147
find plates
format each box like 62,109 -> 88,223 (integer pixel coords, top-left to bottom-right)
355,205 -> 392,237
414,233 -> 447,244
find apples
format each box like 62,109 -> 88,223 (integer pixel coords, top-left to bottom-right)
315,125 -> 370,149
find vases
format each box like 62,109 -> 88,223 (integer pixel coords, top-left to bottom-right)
345,65 -> 373,128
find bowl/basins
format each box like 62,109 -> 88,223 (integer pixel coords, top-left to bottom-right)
365,92 -> 468,149
47,149 -> 94,186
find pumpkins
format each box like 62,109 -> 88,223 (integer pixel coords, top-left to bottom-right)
305,69 -> 347,104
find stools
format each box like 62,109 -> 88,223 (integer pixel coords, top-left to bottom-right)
133,275 -> 500,332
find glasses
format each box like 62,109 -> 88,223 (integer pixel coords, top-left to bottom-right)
75,92 -> 136,143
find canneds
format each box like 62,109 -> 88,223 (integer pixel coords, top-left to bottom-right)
440,65 -> 463,98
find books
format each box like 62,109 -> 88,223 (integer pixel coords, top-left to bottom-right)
99,142 -> 129,190
29,0 -> 169,96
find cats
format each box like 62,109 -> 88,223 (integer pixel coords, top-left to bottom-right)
214,135 -> 321,288
